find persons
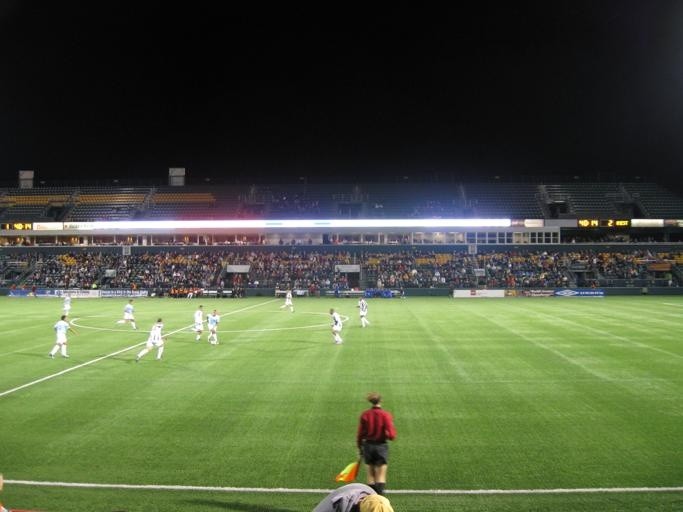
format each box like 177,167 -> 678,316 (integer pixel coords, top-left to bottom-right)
62,296 -> 72,316
206,309 -> 221,344
0,234 -> 679,299
115,299 -> 137,329
279,290 -> 294,312
355,296 -> 370,327
48,315 -> 79,359
356,392 -> 397,494
312,482 -> 395,512
135,317 -> 165,363
191,304 -> 204,340
329,308 -> 342,344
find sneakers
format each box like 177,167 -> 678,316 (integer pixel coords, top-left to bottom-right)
48,352 -> 55,360
61,355 -> 70,358
135,355 -> 140,363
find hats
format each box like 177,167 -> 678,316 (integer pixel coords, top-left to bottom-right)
359,494 -> 394,512
